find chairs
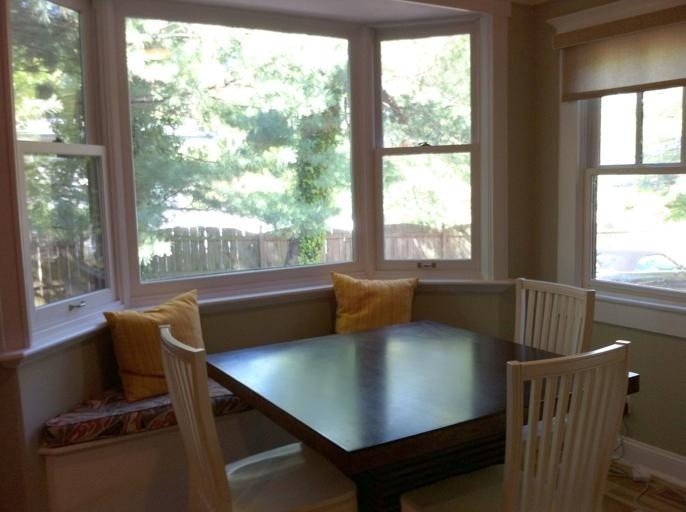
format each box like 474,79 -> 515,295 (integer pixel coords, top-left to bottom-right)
513,277 -> 597,356
400,339 -> 631,512
157,323 -> 355,512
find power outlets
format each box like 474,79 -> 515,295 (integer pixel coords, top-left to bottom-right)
624,395 -> 632,415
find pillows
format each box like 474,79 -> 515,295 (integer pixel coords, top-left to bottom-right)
102,287 -> 204,402
330,271 -> 419,333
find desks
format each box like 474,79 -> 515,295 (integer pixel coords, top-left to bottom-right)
204,322 -> 640,511
